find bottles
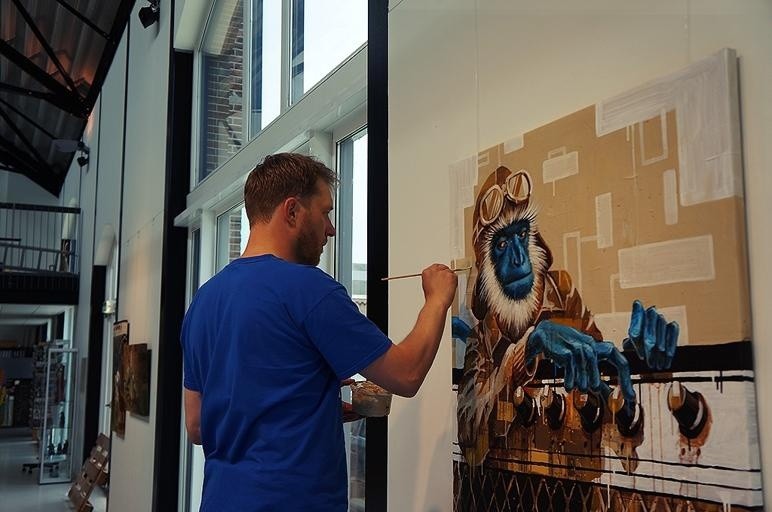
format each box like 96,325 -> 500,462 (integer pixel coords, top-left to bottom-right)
608,387 -> 644,436
569,387 -> 605,430
511,382 -> 535,425
665,383 -> 708,437
538,387 -> 566,430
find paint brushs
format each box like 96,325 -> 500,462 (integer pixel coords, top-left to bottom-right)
381,267 -> 472,281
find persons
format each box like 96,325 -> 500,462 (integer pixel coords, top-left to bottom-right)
177,151 -> 459,511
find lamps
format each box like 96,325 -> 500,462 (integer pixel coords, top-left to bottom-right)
139,0 -> 160,28
76,141 -> 90,167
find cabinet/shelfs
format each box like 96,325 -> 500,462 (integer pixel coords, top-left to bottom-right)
68,433 -> 111,512
31,339 -> 79,485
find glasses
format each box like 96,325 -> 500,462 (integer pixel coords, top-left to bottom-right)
480,169 -> 532,226
